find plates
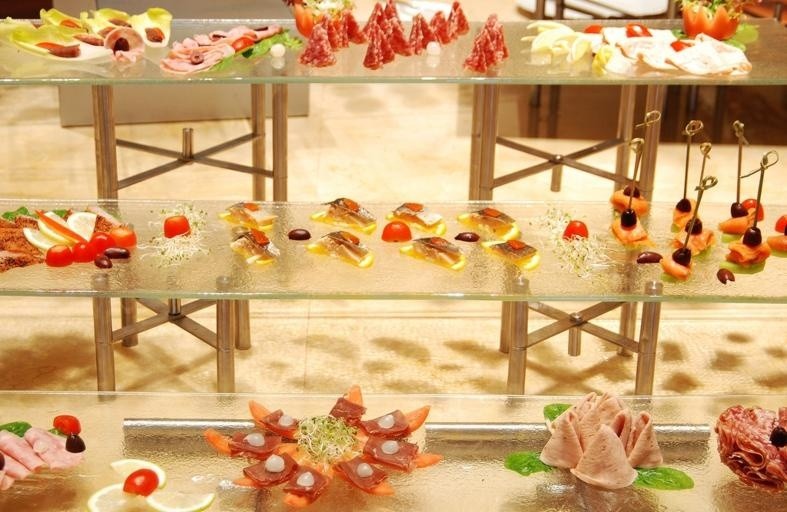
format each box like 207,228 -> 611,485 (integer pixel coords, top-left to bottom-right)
2,11 -> 786,512
